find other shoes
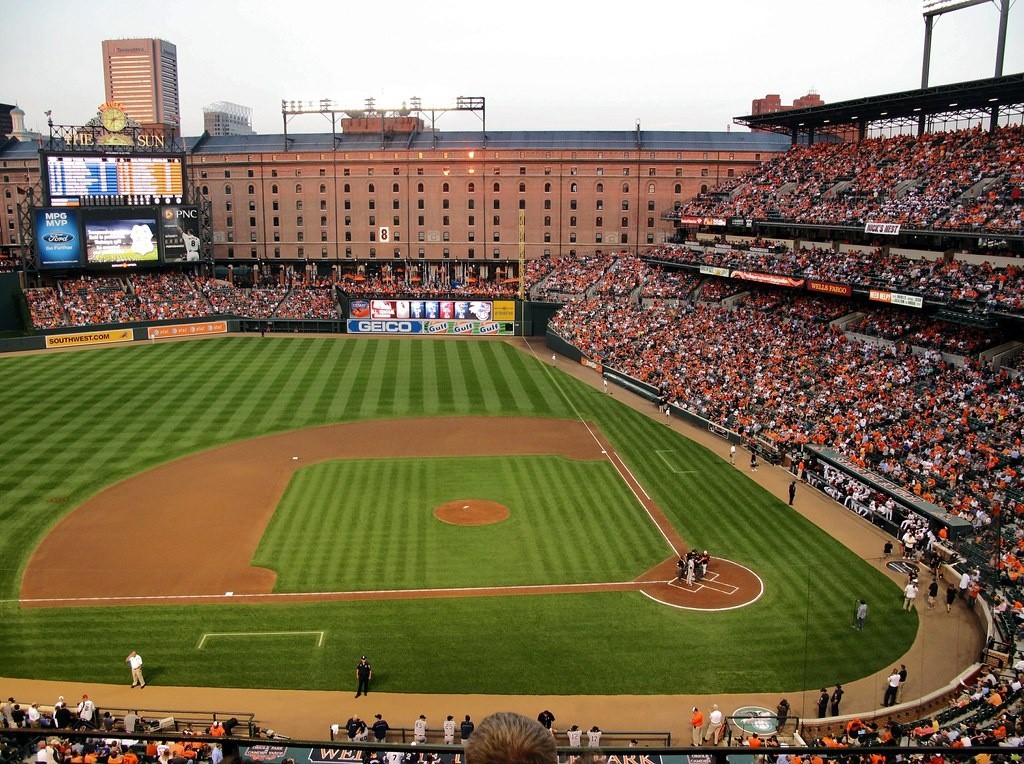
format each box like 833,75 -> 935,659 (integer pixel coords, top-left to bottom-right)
691,743 -> 695,746
364,693 -> 367,696
355,693 -> 360,698
698,743 -> 702,746
141,684 -> 146,689
131,685 -> 137,688
702,737 -> 708,742
714,740 -> 717,746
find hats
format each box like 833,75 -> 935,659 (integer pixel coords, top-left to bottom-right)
374,714 -> 382,719
361,655 -> 367,660
631,739 -> 638,744
446,716 -> 453,721
50,740 -> 58,745
570,725 -> 578,730
466,715 -> 470,722
82,694 -> 88,699
913,579 -> 919,583
40,743 -> 45,749
420,715 -> 426,719
681,549 -> 697,561
820,688 -> 827,692
59,696 -> 64,700
835,683 -> 841,689
692,707 -> 698,712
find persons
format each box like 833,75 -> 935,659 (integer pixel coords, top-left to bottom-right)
126,651 -> 145,689
355,656 -> 372,698
0,122 -> 1024,764
176,226 -> 200,261
789,481 -> 796,505
